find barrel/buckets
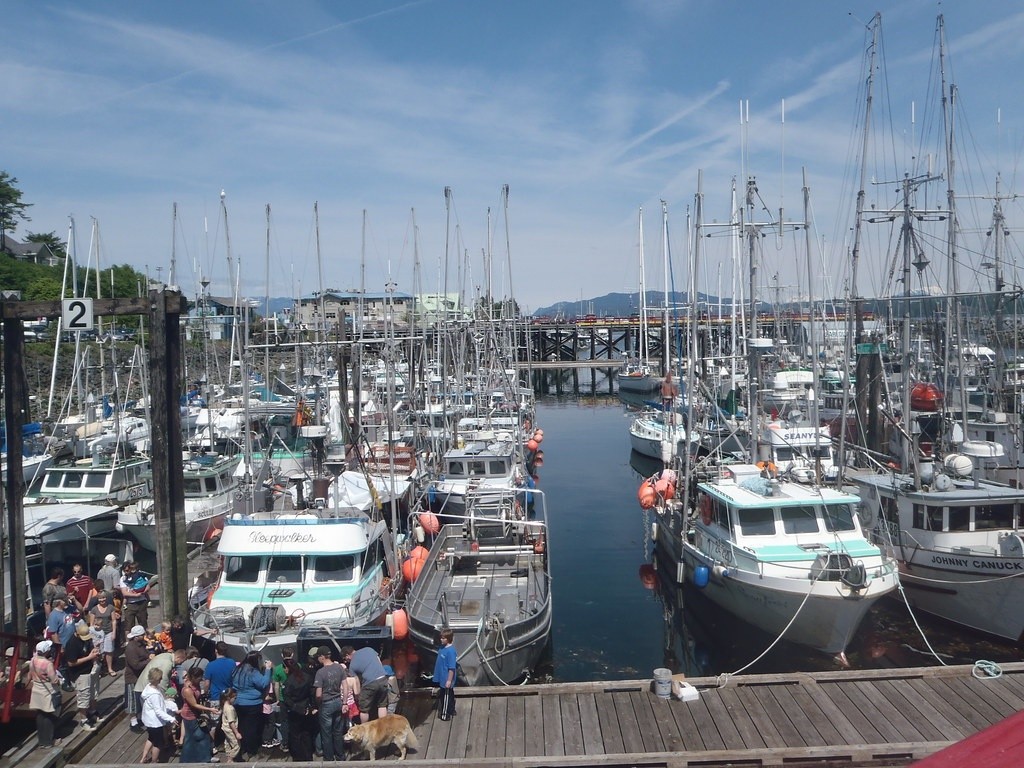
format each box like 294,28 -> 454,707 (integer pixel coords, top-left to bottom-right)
654,667 -> 672,699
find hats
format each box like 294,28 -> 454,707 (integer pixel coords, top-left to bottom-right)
126,625 -> 145,638
53,593 -> 68,600
36,641 -> 53,654
78,624 -> 92,641
313,645 -> 332,657
105,554 -> 117,561
166,688 -> 177,697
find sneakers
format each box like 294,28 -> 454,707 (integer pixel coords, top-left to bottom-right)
80,719 -> 96,731
90,712 -> 104,722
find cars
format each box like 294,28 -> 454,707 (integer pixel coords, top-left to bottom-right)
604,314 -> 615,322
105,329 -> 125,341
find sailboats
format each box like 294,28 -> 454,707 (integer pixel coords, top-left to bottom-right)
0,184 -> 610,691
616,8 -> 1023,670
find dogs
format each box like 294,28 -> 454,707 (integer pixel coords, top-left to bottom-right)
341,713 -> 419,761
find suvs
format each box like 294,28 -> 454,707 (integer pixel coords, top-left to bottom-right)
584,313 -> 598,323
628,312 -> 640,322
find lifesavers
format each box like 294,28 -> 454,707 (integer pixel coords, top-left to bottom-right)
860,501 -> 871,522
701,496 -> 711,525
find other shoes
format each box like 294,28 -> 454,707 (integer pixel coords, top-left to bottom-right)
122,605 -> 127,609
146,602 -> 152,606
279,744 -> 289,752
211,757 -> 220,763
262,738 -> 280,747
213,749 -> 217,754
130,722 -> 139,731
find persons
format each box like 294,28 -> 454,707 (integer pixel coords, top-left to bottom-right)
125,615 -> 219,731
134,645 -> 400,764
30,554 -> 150,748
433,629 -> 457,721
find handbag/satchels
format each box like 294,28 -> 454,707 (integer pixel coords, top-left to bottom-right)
198,711 -> 212,732
51,691 -> 62,708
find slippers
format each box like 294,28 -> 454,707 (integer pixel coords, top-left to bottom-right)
109,670 -> 117,677
